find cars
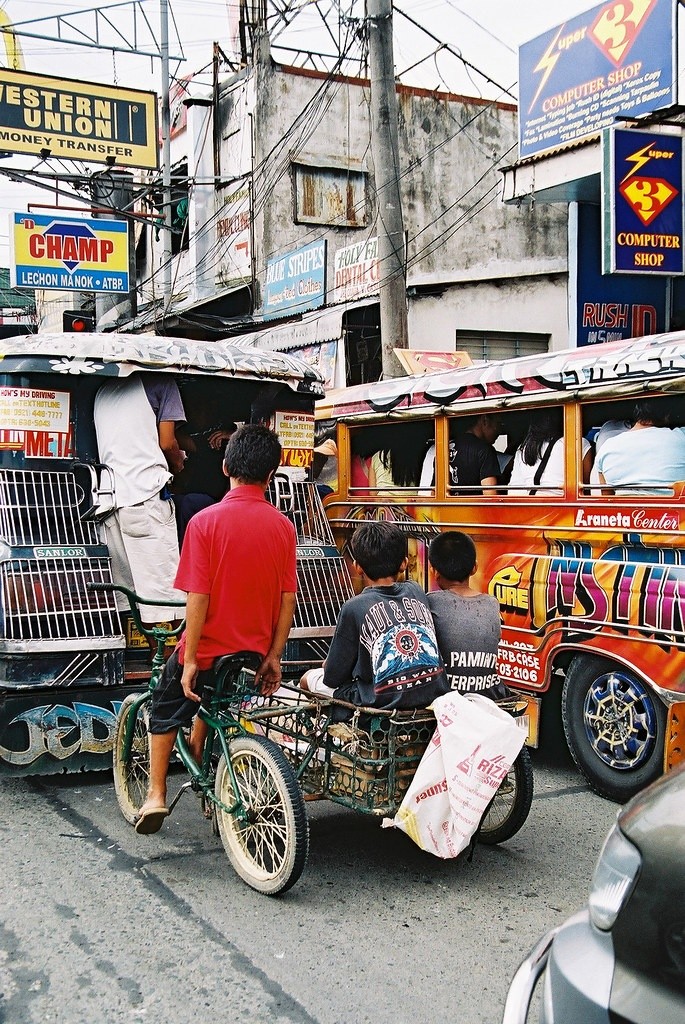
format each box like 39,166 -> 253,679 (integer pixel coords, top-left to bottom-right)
501,766 -> 685,1024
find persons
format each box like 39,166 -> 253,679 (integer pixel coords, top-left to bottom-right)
89,373 -> 246,663
424,529 -> 512,702
300,522 -> 448,736
349,394 -> 685,499
133,425 -> 298,834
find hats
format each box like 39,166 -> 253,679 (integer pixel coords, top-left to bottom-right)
314,439 -> 338,456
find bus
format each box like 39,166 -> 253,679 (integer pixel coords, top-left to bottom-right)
316,328 -> 685,803
0,325 -> 357,778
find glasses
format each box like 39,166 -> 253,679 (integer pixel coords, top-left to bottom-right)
488,420 -> 504,429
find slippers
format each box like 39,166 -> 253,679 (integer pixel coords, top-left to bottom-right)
132,808 -> 169,834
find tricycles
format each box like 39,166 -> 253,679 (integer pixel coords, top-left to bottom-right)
89,579 -> 533,897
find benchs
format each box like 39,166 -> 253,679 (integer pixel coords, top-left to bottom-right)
328,699 -> 528,766
